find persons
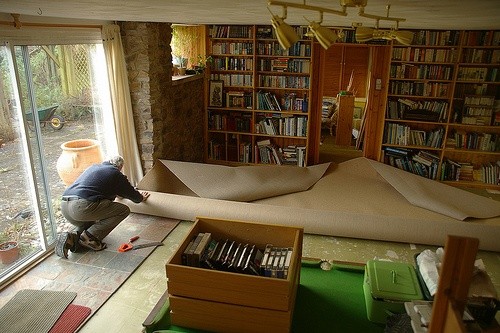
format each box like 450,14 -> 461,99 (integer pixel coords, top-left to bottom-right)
55,155 -> 150,259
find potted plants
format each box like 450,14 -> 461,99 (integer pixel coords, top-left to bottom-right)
171,26 -> 199,76
197,54 -> 213,74
0,241 -> 19,264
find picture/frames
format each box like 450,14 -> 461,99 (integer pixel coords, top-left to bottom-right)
208,80 -> 224,107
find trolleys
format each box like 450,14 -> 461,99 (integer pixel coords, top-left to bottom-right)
25,104 -> 68,130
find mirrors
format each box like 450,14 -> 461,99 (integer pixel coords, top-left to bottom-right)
316,44 -> 373,166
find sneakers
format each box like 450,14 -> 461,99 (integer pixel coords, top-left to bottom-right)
78,231 -> 107,251
55,232 -> 73,260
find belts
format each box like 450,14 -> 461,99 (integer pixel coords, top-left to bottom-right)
62,197 -> 79,201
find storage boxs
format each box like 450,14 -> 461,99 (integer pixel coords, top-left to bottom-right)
165,216 -> 305,333
363,261 -> 423,324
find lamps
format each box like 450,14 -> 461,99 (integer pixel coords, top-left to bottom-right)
266,0 -> 414,51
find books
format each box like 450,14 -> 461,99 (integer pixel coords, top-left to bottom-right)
207,25 -> 500,185
181,233 -> 211,267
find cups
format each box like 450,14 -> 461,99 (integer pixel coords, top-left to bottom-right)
177,67 -> 186,76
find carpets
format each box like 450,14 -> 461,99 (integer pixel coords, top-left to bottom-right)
0,289 -> 91,333
114,157 -> 500,253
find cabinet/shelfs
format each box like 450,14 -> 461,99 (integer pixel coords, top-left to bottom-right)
199,24 -> 500,190
